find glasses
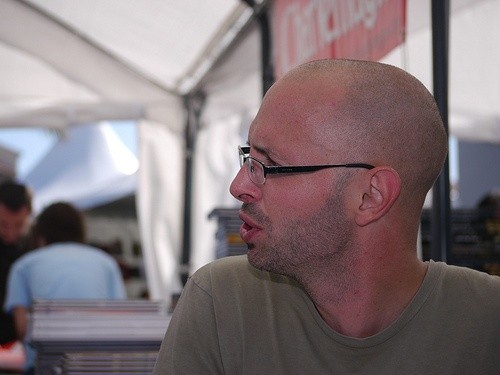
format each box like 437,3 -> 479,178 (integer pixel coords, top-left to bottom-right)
238,145 -> 374,186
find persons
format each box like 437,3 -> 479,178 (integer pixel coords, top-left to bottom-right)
147,58 -> 500,375
3,201 -> 128,372
0,180 -> 36,345
